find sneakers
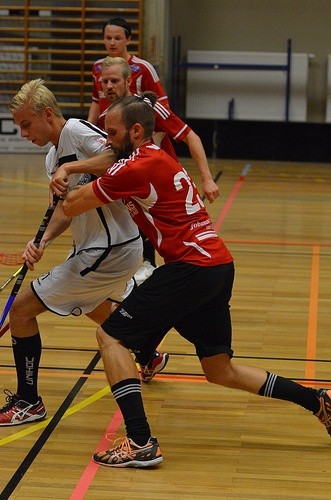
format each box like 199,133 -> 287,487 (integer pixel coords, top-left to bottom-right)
312,388 -> 331,437
135,352 -> 169,384
92,436 -> 164,469
0,389 -> 48,427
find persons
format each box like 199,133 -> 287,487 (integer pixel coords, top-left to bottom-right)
0,78 -> 170,426
88,18 -> 169,134
62,91 -> 331,468
101,56 -> 221,269
